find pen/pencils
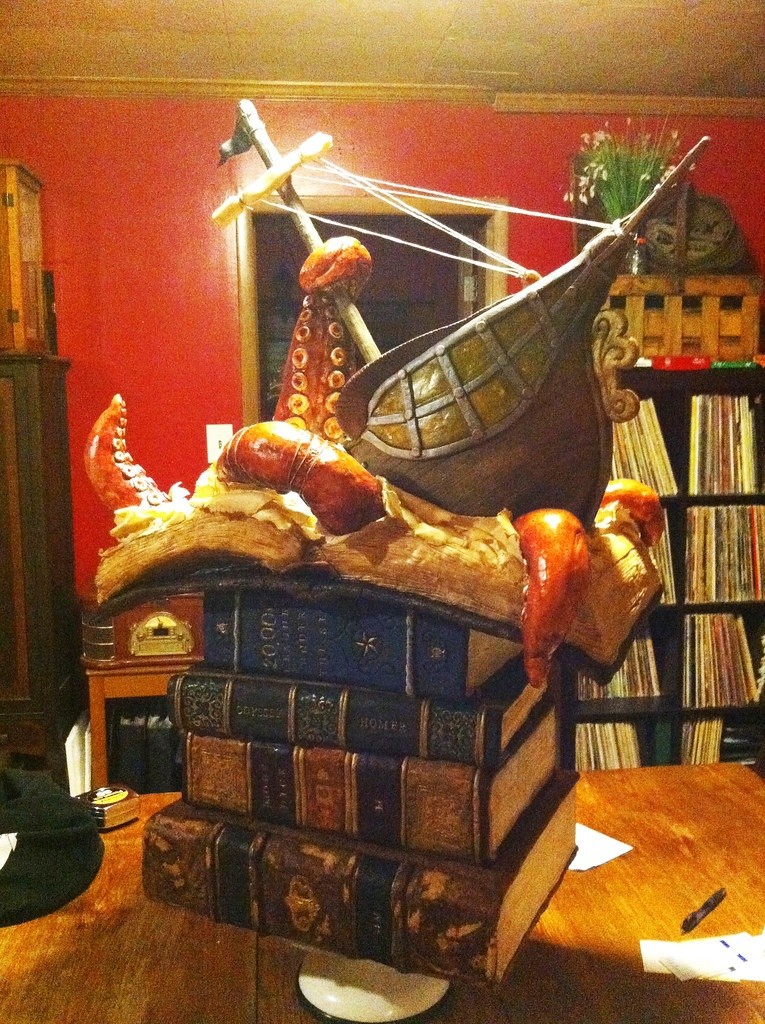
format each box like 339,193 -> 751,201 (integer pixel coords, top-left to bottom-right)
681,887 -> 725,932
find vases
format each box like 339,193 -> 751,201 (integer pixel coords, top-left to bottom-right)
625,241 -> 646,275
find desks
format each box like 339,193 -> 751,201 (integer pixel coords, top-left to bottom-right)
85,663 -> 193,793
0,762 -> 765,1024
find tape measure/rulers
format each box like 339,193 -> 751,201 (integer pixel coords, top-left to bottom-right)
75,785 -> 142,831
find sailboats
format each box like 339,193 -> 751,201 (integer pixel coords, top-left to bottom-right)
211,86 -> 713,531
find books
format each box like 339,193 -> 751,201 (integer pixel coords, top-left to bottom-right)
678,714 -> 723,765
647,509 -> 675,605
574,723 -> 642,772
687,395 -> 765,496
90,493 -> 662,988
607,398 -> 678,498
682,612 -> 760,711
575,623 -> 660,701
685,505 -> 765,603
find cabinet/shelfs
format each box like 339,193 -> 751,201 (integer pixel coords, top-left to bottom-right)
560,368 -> 765,776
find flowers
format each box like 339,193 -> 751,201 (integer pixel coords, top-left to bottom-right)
562,105 -> 696,232
